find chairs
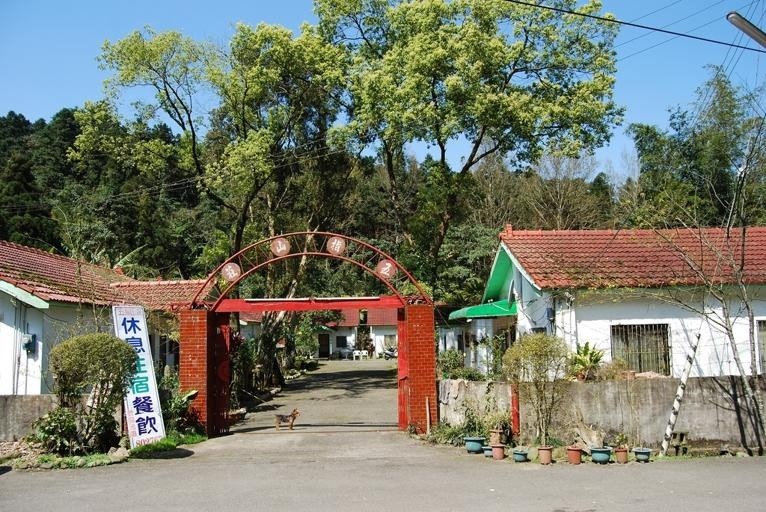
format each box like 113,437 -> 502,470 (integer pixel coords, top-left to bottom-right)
353,350 -> 361,361
361,350 -> 368,361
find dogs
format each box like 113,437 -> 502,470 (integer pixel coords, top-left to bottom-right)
274,408 -> 300,431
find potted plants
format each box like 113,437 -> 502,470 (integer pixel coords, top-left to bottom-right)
462,332 -> 654,465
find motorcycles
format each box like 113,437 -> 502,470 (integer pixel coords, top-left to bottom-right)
383,347 -> 398,361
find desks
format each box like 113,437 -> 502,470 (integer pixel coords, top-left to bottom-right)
341,350 -> 351,360
